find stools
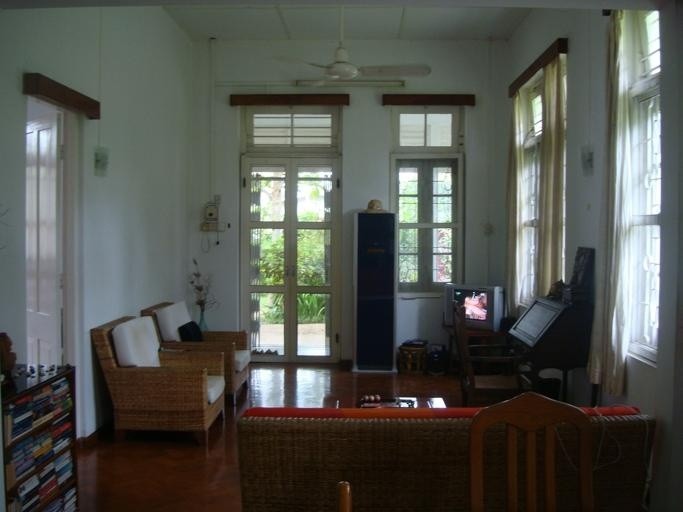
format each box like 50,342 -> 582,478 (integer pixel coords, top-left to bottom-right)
397,343 -> 427,374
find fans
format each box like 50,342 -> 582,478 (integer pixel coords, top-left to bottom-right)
271,6 -> 432,83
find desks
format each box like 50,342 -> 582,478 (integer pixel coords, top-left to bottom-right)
442,324 -> 509,377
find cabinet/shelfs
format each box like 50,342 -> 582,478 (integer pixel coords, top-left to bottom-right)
0,364 -> 79,512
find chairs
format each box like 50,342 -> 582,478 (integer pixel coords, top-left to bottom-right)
451,299 -> 522,408
468,390 -> 597,511
139,301 -> 252,406
90,314 -> 226,440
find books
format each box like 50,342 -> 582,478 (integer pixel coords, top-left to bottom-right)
4,377 -> 77,512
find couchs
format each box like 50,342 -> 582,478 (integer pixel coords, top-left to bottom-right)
236,405 -> 657,512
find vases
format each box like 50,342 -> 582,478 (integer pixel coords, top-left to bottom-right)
196,312 -> 207,329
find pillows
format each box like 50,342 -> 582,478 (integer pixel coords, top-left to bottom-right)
108,316 -> 161,368
176,319 -> 204,343
151,299 -> 191,343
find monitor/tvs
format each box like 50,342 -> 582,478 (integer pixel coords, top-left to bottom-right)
444,282 -> 505,333
